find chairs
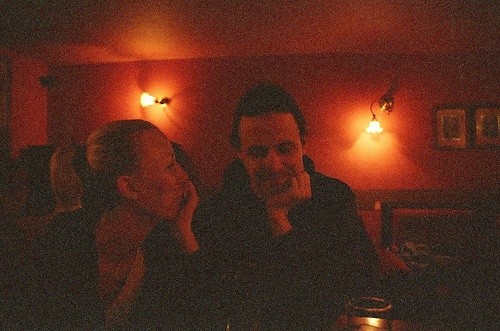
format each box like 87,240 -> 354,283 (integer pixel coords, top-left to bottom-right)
379,200 -> 482,261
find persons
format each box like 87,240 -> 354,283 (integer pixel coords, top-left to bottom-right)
191,80 -> 379,331
0,118 -> 243,331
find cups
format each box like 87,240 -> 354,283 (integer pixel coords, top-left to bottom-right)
348,297 -> 393,331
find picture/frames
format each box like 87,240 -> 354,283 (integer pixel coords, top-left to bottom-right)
431,103 -> 500,151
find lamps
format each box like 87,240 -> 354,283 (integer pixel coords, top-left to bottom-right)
140,92 -> 169,107
366,94 -> 395,134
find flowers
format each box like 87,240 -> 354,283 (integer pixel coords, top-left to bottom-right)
386,240 -> 428,269
